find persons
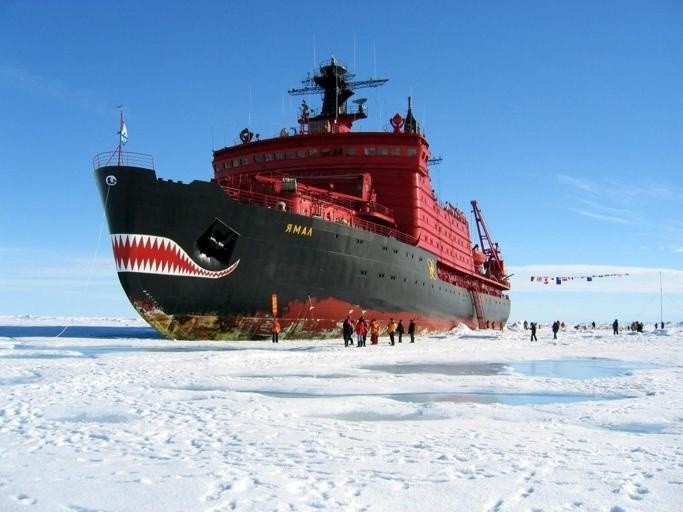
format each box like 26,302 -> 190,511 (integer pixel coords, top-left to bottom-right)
269,318 -> 280,343
527,322 -> 537,341
369,318 -> 380,345
523,320 -> 527,330
552,321 -> 558,339
358,315 -> 367,346
590,321 -> 595,329
387,317 -> 396,346
396,319 -> 404,343
612,319 -> 618,335
407,319 -> 415,343
491,321 -> 494,329
343,319 -> 352,348
626,320 -> 664,333
485,319 -> 489,329
499,322 -> 503,331
346,315 -> 354,345
355,319 -> 365,347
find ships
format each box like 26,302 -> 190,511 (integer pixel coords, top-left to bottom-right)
92,55 -> 511,341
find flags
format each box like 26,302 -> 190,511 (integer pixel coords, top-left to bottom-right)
116,110 -> 127,146
531,277 -> 598,285
